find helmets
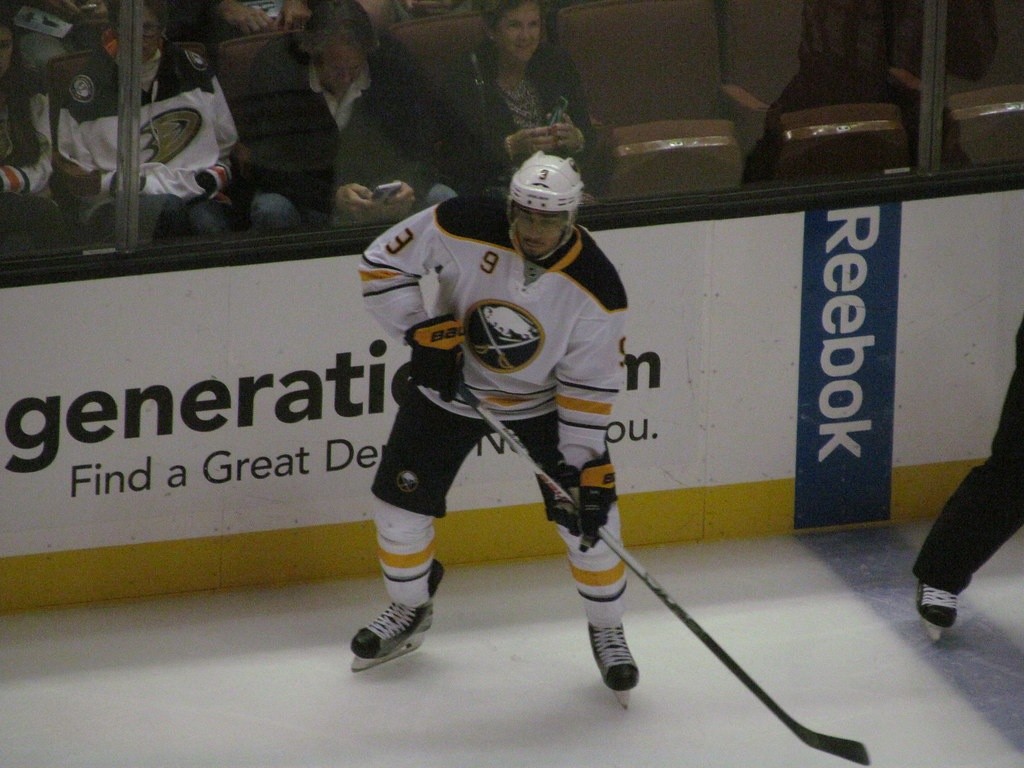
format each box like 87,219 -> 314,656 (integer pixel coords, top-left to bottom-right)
511,151 -> 584,242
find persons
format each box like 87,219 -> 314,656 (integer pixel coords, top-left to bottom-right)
350,151 -> 640,691
913,315 -> 1024,629
738,1 -> 997,197
1,0 -> 602,259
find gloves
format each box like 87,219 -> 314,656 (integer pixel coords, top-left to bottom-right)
546,452 -> 618,552
405,313 -> 466,402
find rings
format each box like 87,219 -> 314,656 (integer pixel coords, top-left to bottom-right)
532,143 -> 537,151
287,20 -> 291,24
559,139 -> 562,146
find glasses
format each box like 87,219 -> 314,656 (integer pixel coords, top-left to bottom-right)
143,25 -> 163,38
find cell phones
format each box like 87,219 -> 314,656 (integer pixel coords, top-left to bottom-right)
371,181 -> 402,202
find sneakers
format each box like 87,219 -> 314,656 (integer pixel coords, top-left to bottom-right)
588,622 -> 639,709
351,559 -> 444,673
916,580 -> 958,640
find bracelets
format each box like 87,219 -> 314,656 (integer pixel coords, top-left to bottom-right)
506,135 -> 515,162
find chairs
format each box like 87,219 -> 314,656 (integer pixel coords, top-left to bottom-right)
216,0 -> 1024,203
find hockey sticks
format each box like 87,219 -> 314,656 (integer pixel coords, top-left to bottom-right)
460,383 -> 873,768
